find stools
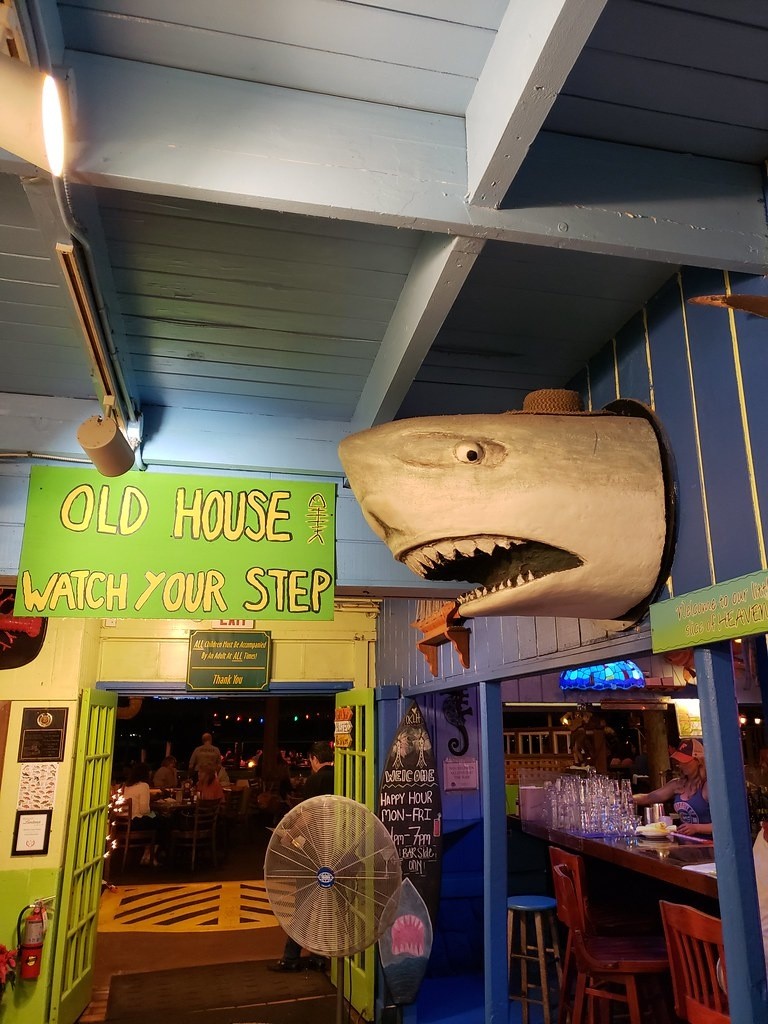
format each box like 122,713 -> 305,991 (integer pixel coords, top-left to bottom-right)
507,895 -> 563,1024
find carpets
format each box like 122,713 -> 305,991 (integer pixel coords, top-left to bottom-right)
104,956 -> 336,1024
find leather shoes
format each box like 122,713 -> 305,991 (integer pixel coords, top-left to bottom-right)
307,955 -> 326,972
267,959 -> 300,972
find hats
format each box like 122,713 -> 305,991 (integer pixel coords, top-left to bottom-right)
670,738 -> 703,763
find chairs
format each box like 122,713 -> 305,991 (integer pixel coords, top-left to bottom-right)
548,846 -> 667,1024
108,798 -> 158,869
659,899 -> 732,1024
218,787 -> 265,840
167,797 -> 223,864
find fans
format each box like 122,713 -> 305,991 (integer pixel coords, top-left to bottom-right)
263,794 -> 403,1024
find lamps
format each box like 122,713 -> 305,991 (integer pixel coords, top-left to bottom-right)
0,51 -> 66,178
75,395 -> 135,477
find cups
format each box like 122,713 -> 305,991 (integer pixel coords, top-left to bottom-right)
541,774 -> 665,846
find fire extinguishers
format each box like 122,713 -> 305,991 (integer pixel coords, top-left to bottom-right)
15,896 -> 57,980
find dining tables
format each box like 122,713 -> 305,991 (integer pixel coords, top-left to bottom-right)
147,786 -> 201,832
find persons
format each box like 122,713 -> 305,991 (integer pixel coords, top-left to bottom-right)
631,739 -> 713,841
111,734 -> 334,973
